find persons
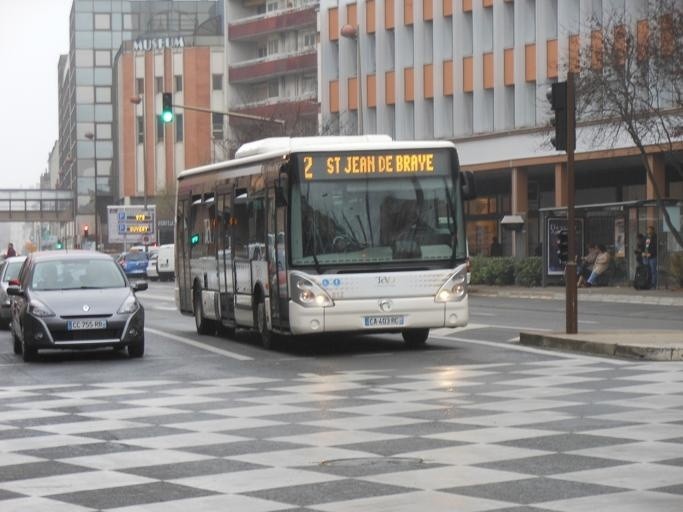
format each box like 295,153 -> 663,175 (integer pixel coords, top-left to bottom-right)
4,243 -> 16,258
634,226 -> 657,290
564,243 -> 610,288
491,236 -> 499,257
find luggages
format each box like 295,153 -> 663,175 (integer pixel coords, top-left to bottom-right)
634,255 -> 653,289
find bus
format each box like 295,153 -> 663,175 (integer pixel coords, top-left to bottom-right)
174,134 -> 472,345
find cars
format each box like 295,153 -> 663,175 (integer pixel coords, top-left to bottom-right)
113,244 -> 175,280
0,249 -> 148,361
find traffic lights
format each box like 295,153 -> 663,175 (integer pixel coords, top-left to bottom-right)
57,244 -> 62,249
163,92 -> 173,124
85,225 -> 88,238
